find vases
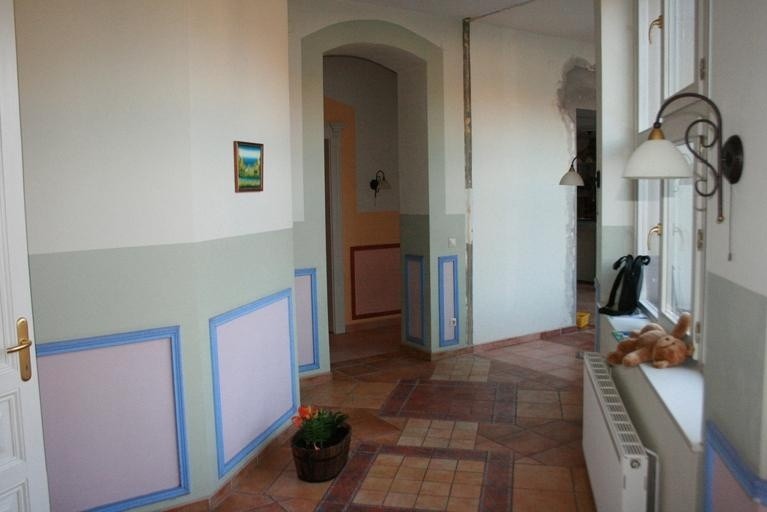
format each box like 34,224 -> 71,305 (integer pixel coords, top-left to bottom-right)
290,422 -> 351,483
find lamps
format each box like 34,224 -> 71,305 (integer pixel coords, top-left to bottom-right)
370,169 -> 392,197
623,92 -> 745,224
559,153 -> 601,190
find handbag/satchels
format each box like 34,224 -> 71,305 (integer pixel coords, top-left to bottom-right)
599,256 -> 650,316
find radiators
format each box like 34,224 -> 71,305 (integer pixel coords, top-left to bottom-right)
581,349 -> 661,512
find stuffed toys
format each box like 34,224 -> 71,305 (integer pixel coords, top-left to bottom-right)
606,311 -> 696,369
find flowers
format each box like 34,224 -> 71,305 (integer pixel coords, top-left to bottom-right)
290,405 -> 348,444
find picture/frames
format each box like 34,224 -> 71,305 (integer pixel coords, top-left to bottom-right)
234,140 -> 263,193
